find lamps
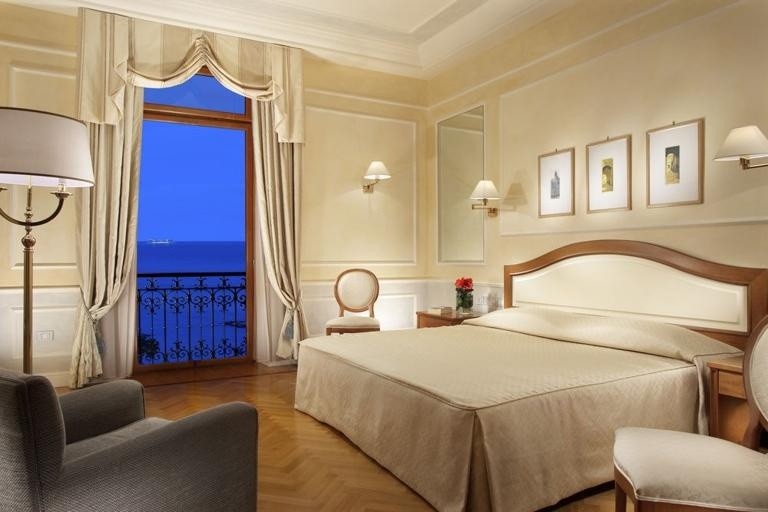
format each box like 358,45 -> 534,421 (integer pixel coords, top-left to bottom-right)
363,161 -> 390,193
713,124 -> 768,170
470,180 -> 501,218
1,105 -> 95,373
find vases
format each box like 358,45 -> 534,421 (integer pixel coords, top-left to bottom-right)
455,288 -> 474,313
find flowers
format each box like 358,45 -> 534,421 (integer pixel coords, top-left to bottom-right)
456,278 -> 473,310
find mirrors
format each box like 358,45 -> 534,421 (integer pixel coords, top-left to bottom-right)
436,103 -> 486,265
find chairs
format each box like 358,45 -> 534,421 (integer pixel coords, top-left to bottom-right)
0,366 -> 259,512
325,269 -> 380,336
611,314 -> 768,512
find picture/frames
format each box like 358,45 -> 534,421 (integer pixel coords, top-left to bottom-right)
646,117 -> 705,209
585,133 -> 632,214
538,146 -> 575,218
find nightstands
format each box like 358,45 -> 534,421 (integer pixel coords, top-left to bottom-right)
416,310 -> 480,328
708,355 -> 768,454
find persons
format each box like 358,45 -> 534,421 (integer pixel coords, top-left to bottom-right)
550,170 -> 562,198
665,146 -> 680,184
599,158 -> 614,192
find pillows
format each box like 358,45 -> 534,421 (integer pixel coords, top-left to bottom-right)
461,307 -> 744,435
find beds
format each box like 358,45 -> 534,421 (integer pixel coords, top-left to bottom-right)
293,240 -> 768,512
